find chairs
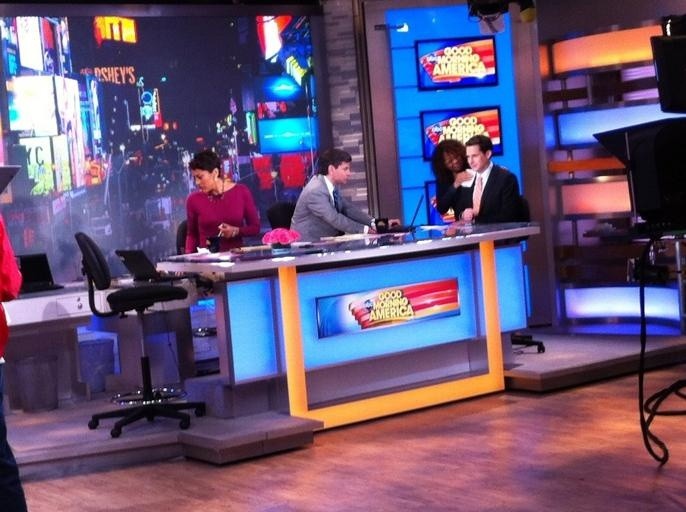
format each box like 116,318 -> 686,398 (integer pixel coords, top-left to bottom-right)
75,220 -> 206,438
507,195 -> 546,353
266,201 -> 296,232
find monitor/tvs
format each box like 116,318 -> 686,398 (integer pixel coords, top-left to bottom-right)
256,115 -> 320,154
256,74 -> 306,101
414,34 -> 499,91
425,179 -> 455,225
585,67 -> 625,107
420,104 -> 504,161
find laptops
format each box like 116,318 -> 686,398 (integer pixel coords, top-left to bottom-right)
389,194 -> 425,233
114,249 -> 197,282
15,252 -> 65,294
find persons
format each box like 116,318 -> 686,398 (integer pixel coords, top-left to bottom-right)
288,147 -> 402,243
1,212 -> 34,512
181,150 -> 263,256
431,138 -> 509,229
428,135 -> 520,224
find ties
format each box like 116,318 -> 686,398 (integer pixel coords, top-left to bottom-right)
473,176 -> 482,215
332,189 -> 340,211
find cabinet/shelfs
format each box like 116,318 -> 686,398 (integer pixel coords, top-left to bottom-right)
625,229 -> 686,468
